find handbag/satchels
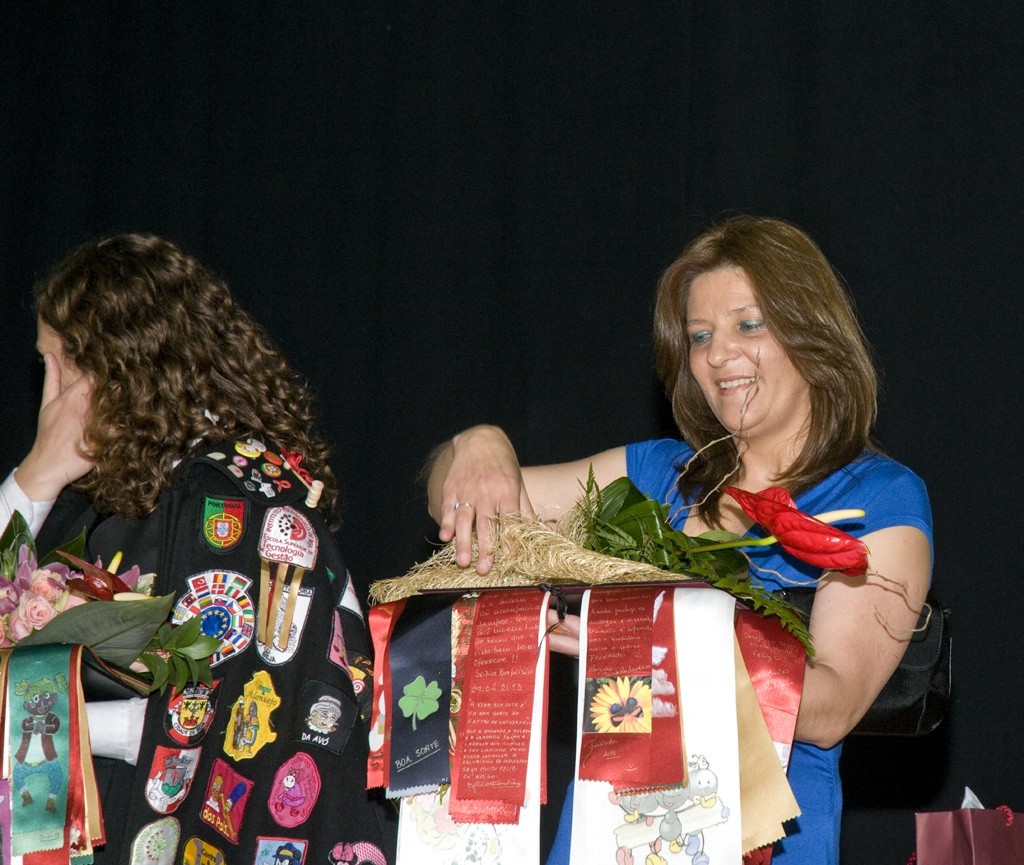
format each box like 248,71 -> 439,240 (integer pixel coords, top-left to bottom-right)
769,588 -> 952,738
907,806 -> 1024,865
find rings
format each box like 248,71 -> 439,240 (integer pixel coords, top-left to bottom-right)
454,502 -> 474,509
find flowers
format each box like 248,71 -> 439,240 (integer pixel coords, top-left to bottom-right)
0,509 -> 223,698
369,354 -> 934,668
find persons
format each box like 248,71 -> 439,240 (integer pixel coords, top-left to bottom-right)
430,216 -> 935,865
0,238 -> 399,865
